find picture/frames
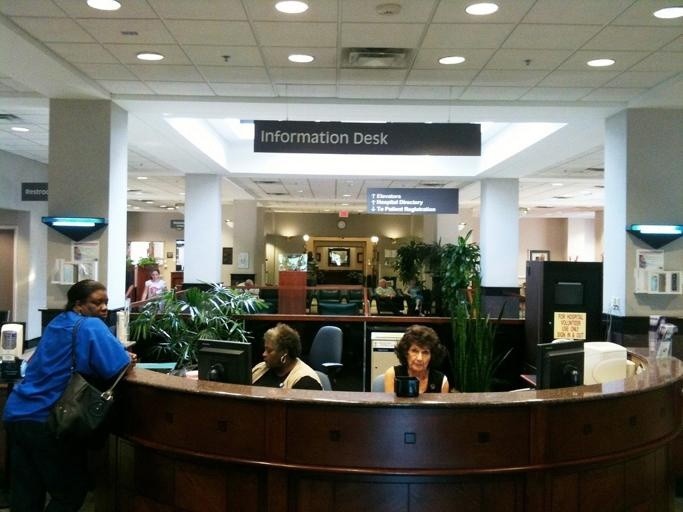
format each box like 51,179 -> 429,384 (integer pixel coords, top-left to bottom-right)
529,250 -> 550,263
328,247 -> 350,267
357,252 -> 364,263
315,253 -> 321,261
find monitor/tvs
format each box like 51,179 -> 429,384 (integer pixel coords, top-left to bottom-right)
535,338 -> 588,389
196,338 -> 252,386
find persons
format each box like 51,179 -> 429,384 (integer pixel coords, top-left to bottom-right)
141,269 -> 168,303
235,279 -> 254,291
403,280 -> 425,316
375,278 -> 407,316
252,323 -> 324,389
0,279 -> 138,511
384,324 -> 449,393
126,269 -> 134,302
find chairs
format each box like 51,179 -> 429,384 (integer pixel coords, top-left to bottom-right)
367,287 -> 439,316
302,325 -> 345,390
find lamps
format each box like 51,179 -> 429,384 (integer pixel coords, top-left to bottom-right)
626,224 -> 682,250
41,217 -> 108,242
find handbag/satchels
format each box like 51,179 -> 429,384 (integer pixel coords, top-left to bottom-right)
47,373 -> 114,444
